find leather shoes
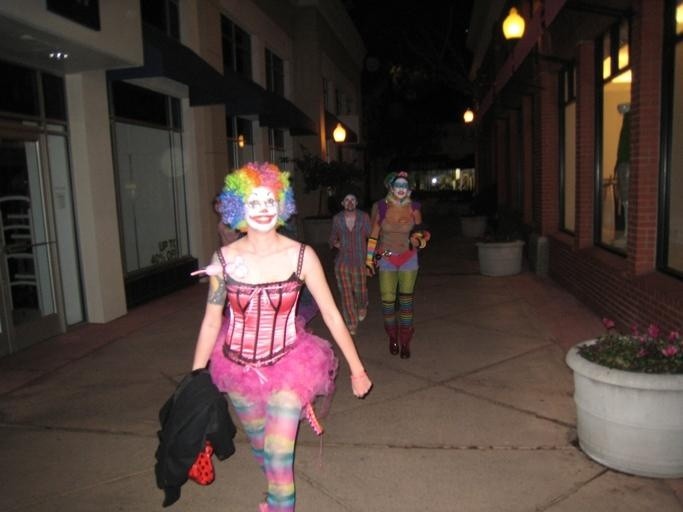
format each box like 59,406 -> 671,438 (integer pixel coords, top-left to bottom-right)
400,341 -> 410,358
390,336 -> 399,355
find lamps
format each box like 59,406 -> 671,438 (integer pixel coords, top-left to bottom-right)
463,107 -> 474,124
501,0 -> 525,40
333,123 -> 347,143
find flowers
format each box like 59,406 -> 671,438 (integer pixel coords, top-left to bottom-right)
580,317 -> 681,374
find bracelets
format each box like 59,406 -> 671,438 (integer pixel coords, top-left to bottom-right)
348,370 -> 369,380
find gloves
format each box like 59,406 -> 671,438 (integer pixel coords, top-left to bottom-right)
366,237 -> 377,277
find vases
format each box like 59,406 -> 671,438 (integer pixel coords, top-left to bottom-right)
476,241 -> 527,278
460,215 -> 487,239
566,333 -> 681,481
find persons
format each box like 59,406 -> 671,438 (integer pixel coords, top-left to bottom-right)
363,171 -> 431,358
191,159 -> 372,511
328,192 -> 373,337
